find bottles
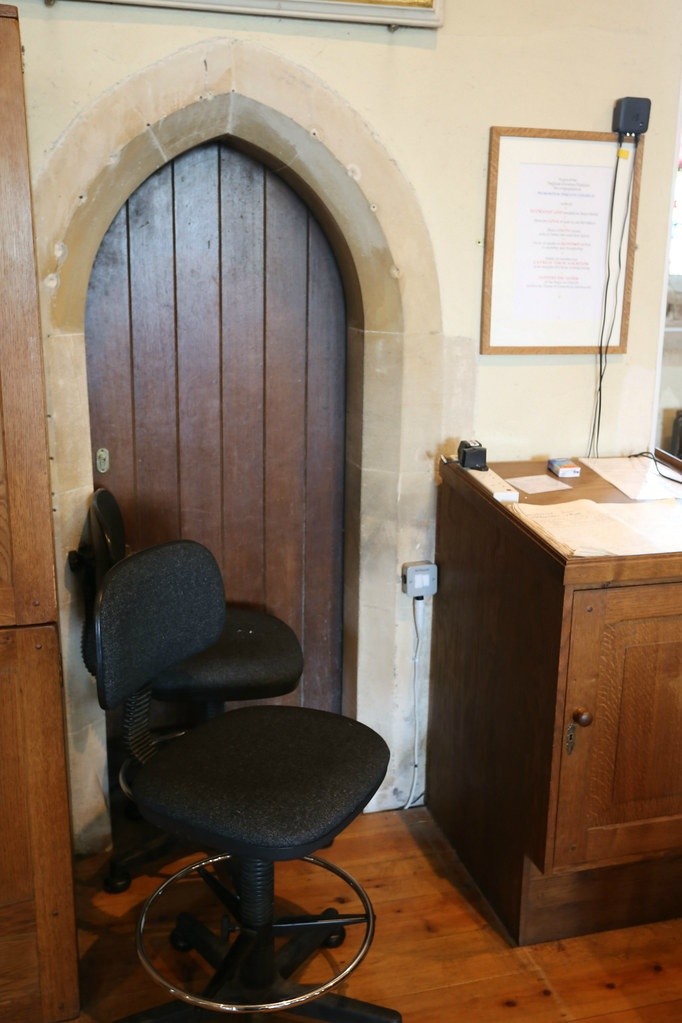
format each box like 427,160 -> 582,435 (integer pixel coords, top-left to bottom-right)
669,411 -> 682,459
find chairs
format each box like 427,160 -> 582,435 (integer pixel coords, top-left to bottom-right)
86,538 -> 402,1023
68,488 -> 302,951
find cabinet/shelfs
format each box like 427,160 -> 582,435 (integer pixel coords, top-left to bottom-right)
422,449 -> 682,948
